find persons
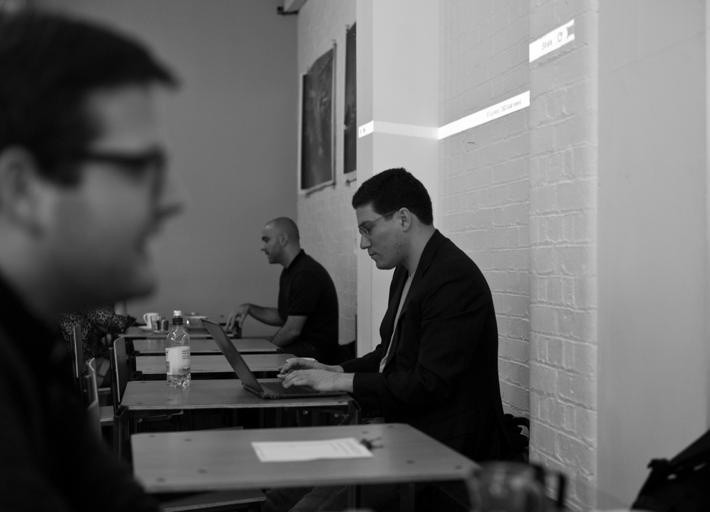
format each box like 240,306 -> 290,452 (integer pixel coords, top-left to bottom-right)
225,216 -> 338,363
277,167 -> 519,511
0,11 -> 191,510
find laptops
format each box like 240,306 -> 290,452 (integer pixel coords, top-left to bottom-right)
200,316 -> 349,399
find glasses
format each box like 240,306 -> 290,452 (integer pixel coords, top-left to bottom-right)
358,209 -> 397,236
41,142 -> 172,183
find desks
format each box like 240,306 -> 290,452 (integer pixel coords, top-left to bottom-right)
73,303 -> 568,512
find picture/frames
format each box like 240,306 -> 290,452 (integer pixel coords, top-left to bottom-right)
297,22 -> 357,196
